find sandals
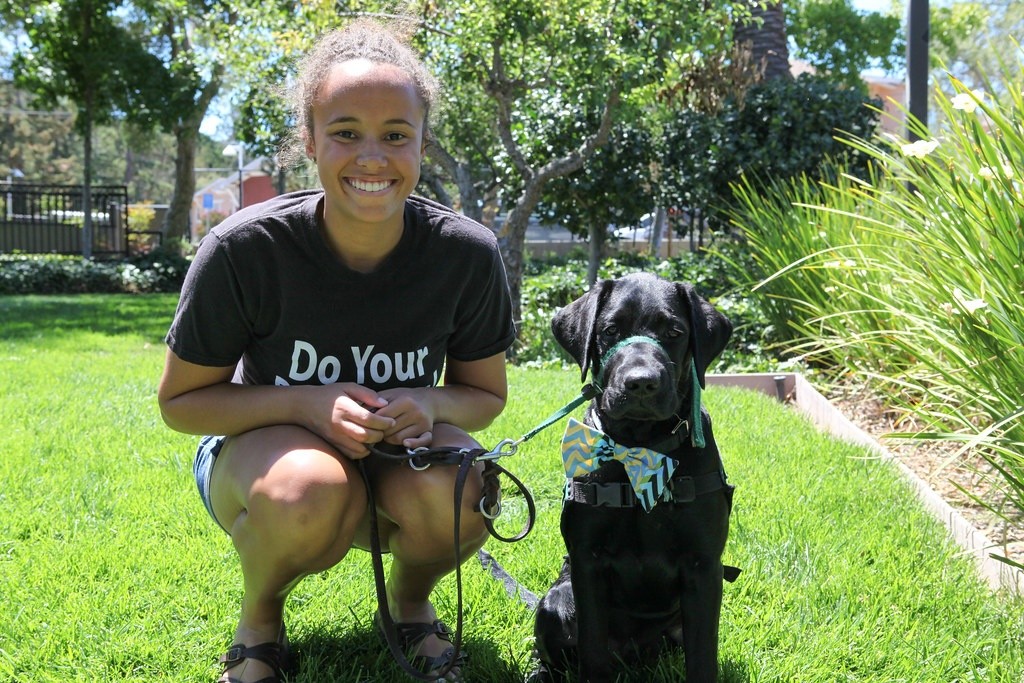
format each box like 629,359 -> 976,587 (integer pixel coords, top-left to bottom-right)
217,622 -> 292,683
372,610 -> 467,683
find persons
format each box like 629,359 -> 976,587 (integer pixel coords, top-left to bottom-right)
159,19 -> 517,683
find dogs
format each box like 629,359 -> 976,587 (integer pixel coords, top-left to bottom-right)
477,273 -> 733,683
